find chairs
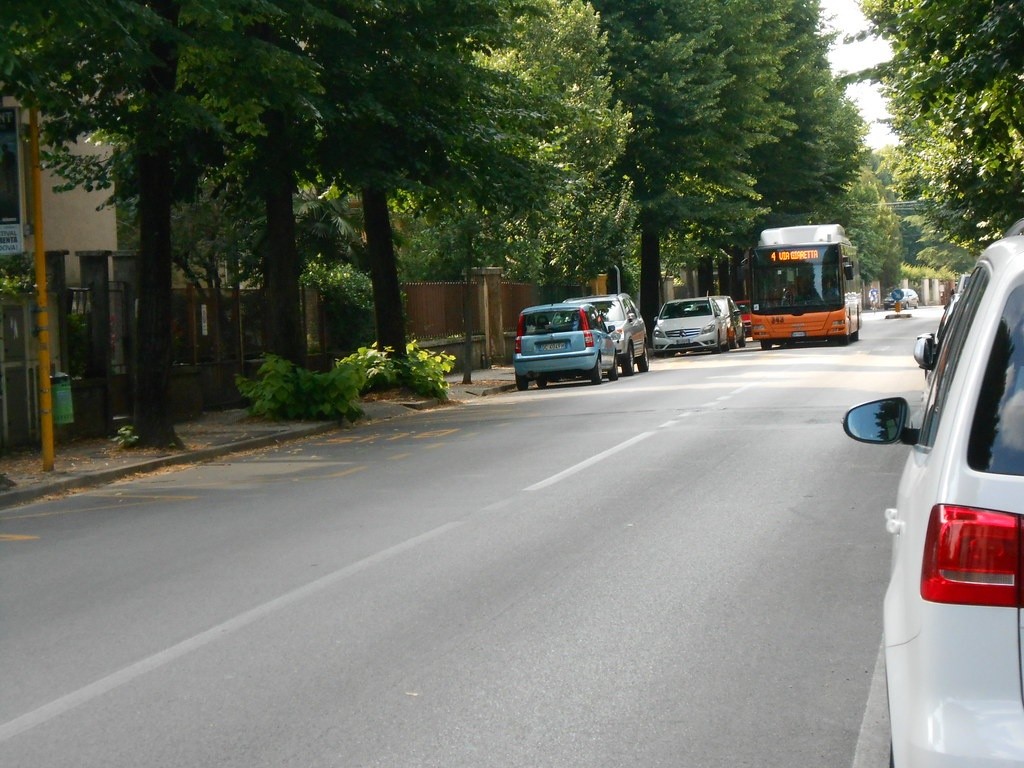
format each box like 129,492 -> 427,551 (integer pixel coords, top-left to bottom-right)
536,316 -> 548,328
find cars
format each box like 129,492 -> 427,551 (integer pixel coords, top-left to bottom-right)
914,274 -> 972,376
883,289 -> 919,310
547,293 -> 649,376
513,303 -> 618,391
736,300 -> 752,337
652,297 -> 730,358
842,217 -> 1024,768
684,296 -> 746,349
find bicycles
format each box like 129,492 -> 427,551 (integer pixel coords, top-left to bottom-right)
869,293 -> 880,313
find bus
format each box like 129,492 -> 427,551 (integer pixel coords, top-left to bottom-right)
740,223 -> 863,351
